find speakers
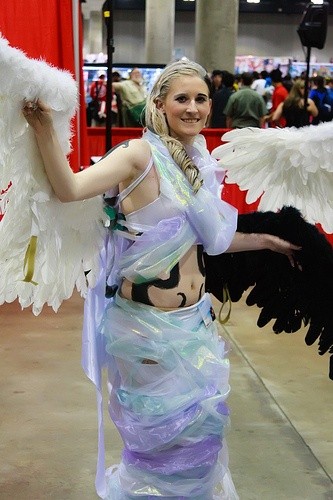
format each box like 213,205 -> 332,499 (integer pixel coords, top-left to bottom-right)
298,2 -> 329,49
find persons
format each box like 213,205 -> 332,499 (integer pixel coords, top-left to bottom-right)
89,67 -> 333,128
22,60 -> 306,500
